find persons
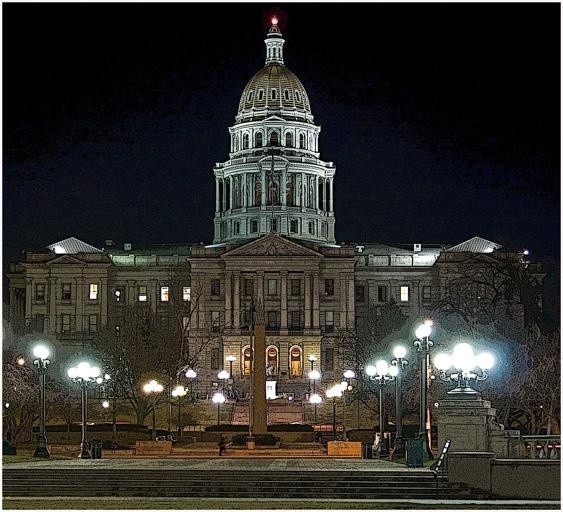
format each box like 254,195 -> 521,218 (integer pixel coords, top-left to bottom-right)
217,436 -> 225,456
539,445 -> 546,458
550,444 -> 558,458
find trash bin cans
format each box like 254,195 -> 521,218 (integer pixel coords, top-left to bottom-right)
91,442 -> 102,458
365,443 -> 372,458
404,438 -> 424,468
